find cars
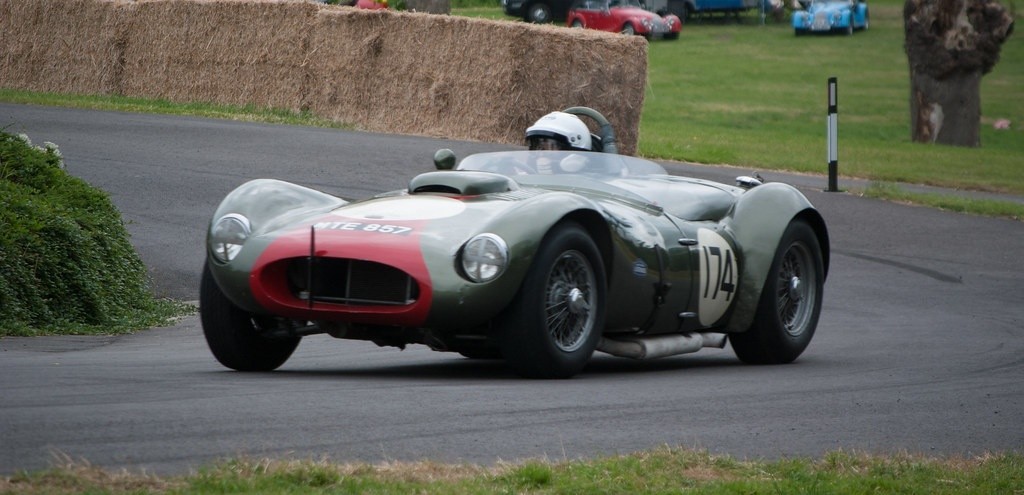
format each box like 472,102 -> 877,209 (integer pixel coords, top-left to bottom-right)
197,106 -> 830,379
566,4 -> 681,40
502,0 -> 584,24
793,0 -> 869,37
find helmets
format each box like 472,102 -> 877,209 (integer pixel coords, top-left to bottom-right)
524,110 -> 591,173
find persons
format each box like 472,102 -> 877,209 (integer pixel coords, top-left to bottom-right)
524,111 -> 591,172
